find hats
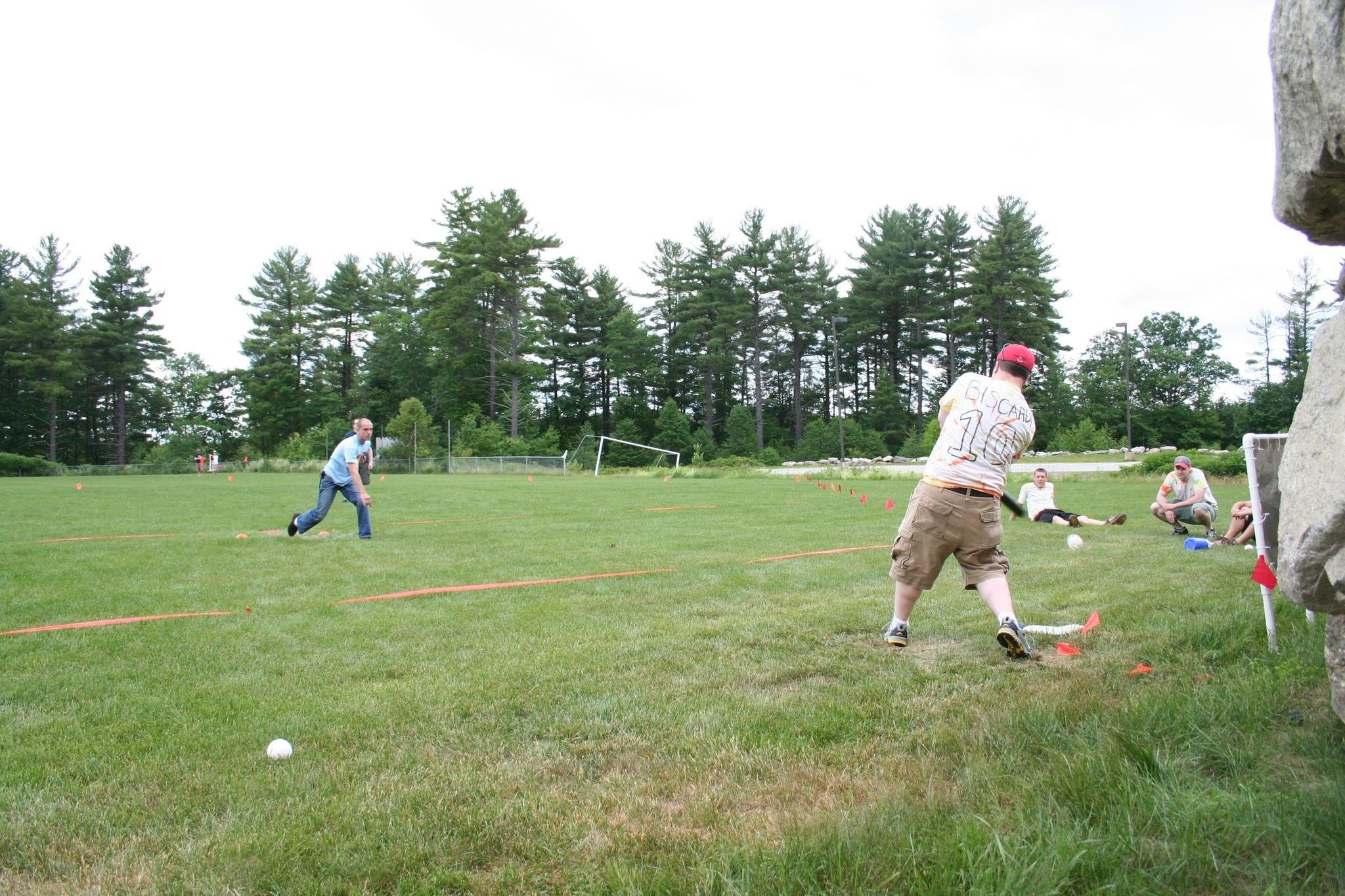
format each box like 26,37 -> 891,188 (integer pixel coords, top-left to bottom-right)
1174,456 -> 1191,469
998,344 -> 1036,372
213,450 -> 216,452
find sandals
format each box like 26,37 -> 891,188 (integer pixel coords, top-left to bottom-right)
1213,536 -> 1229,545
1222,538 -> 1236,546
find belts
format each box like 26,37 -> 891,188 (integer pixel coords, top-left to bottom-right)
944,488 -> 998,498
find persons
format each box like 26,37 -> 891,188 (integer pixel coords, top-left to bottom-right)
1008,469 -> 1127,527
341,419 -> 364,502
1148,454 -> 1219,538
882,342 -> 1039,657
193,448 -> 206,476
287,418 -> 382,541
1209,500 -> 1256,547
209,450 -> 220,475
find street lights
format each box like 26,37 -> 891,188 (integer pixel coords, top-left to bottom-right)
1115,322 -> 1131,452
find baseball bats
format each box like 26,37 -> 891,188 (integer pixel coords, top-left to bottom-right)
1001,492 -> 1024,517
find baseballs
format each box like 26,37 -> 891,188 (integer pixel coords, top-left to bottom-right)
267,739 -> 292,761
1066,534 -> 1083,551
1244,544 -> 1253,550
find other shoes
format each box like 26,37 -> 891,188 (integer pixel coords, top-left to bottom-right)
343,499 -> 346,502
359,537 -> 371,538
287,512 -> 300,536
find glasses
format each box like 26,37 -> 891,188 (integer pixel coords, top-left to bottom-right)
1002,343 -> 1010,361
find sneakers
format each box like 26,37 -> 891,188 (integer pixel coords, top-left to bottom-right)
1204,529 -> 1216,539
882,617 -> 909,646
1105,513 -> 1127,525
1171,526 -> 1189,536
996,616 -> 1034,660
1069,515 -> 1082,528
